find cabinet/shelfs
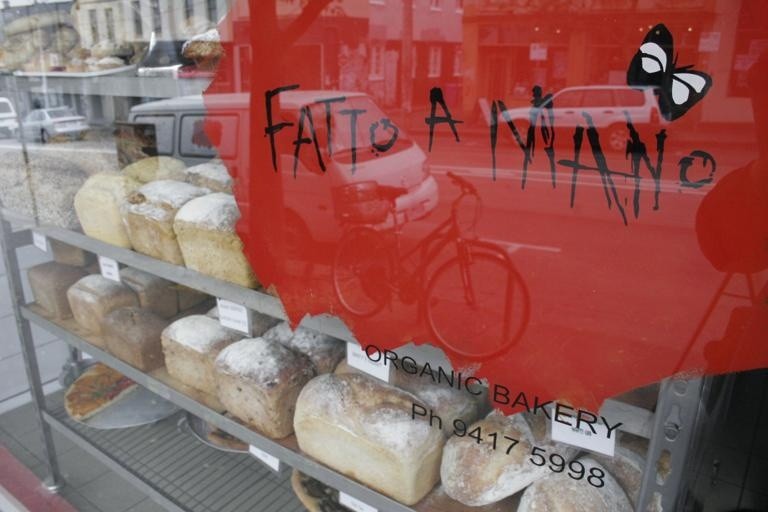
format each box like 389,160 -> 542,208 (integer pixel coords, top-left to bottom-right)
0,72 -> 732,512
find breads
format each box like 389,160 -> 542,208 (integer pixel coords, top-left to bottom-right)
0,0 -> 670,512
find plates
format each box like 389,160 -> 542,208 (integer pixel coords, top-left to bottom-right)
185,410 -> 252,455
65,362 -> 182,431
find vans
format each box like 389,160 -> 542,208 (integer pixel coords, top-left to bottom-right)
123,89 -> 442,235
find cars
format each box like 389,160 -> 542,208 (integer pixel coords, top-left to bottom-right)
16,106 -> 92,147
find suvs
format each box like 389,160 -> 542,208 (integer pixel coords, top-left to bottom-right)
0,97 -> 20,138
483,82 -> 673,152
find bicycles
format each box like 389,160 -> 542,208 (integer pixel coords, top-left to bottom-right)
329,164 -> 533,363
